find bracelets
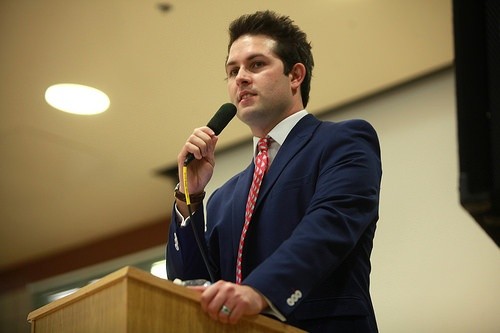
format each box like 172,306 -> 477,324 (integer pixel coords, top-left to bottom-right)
174,182 -> 206,203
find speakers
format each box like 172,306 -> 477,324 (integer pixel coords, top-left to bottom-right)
452,0 -> 500,246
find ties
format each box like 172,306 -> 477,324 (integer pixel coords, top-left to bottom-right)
236,137 -> 273,285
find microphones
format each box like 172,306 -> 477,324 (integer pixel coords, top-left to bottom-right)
183,103 -> 237,165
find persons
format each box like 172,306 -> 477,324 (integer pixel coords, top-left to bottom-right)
166,10 -> 382,333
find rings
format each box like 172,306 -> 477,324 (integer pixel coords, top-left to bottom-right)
222,305 -> 232,315
